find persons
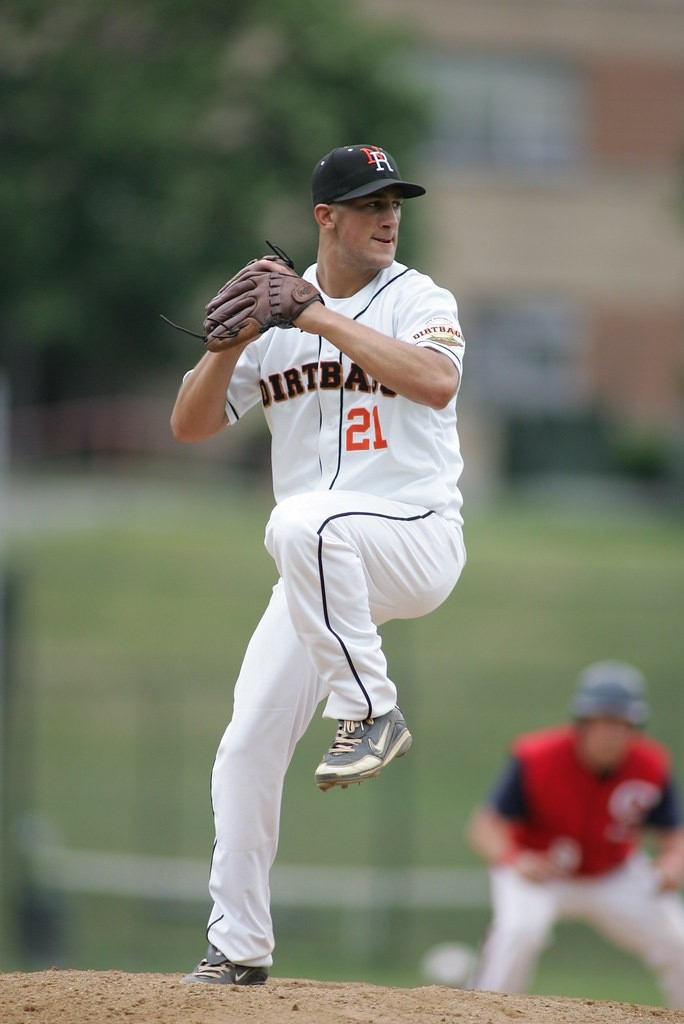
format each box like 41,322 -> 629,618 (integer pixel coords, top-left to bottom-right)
168,141 -> 466,987
422,659 -> 683,1009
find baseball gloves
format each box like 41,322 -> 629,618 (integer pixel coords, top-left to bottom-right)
201,253 -> 325,354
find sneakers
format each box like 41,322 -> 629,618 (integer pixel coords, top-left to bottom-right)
181,943 -> 267,987
314,708 -> 413,791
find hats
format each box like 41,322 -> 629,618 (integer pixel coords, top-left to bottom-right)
572,660 -> 650,725
313,141 -> 425,205
